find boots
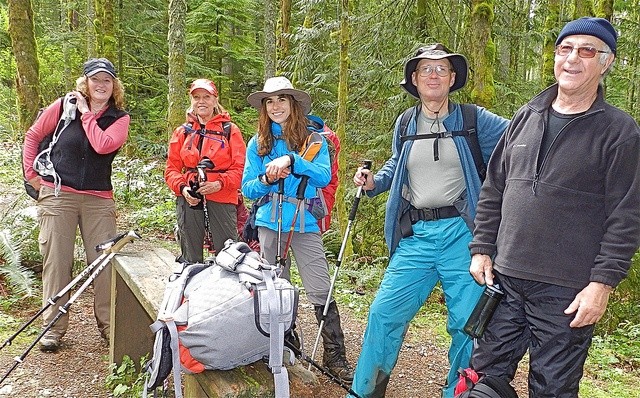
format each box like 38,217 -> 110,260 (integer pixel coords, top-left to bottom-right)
314,300 -> 355,384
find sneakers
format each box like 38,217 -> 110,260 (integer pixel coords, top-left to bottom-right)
101,326 -> 110,342
39,332 -> 61,352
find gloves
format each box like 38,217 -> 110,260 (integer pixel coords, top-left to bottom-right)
186,180 -> 202,210
242,202 -> 260,243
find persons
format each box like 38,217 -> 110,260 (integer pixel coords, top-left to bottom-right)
241,76 -> 356,384
469,18 -> 640,398
345,43 -> 512,398
164,79 -> 246,264
22,57 -> 130,351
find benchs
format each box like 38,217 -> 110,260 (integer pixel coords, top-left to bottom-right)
110,247 -> 321,398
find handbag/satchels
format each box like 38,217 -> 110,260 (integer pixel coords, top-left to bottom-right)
269,192 -> 325,220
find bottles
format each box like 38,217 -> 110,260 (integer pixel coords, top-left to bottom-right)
463,284 -> 505,339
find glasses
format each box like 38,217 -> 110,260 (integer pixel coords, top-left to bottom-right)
413,65 -> 454,77
554,44 -> 610,58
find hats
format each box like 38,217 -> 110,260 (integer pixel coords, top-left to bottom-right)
246,76 -> 311,116
190,79 -> 218,97
83,58 -> 117,79
399,43 -> 468,101
554,17 -> 617,55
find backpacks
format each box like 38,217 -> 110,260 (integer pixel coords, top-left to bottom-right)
22,92 -> 78,201
141,238 -> 303,398
457,367 -> 519,398
259,121 -> 340,234
